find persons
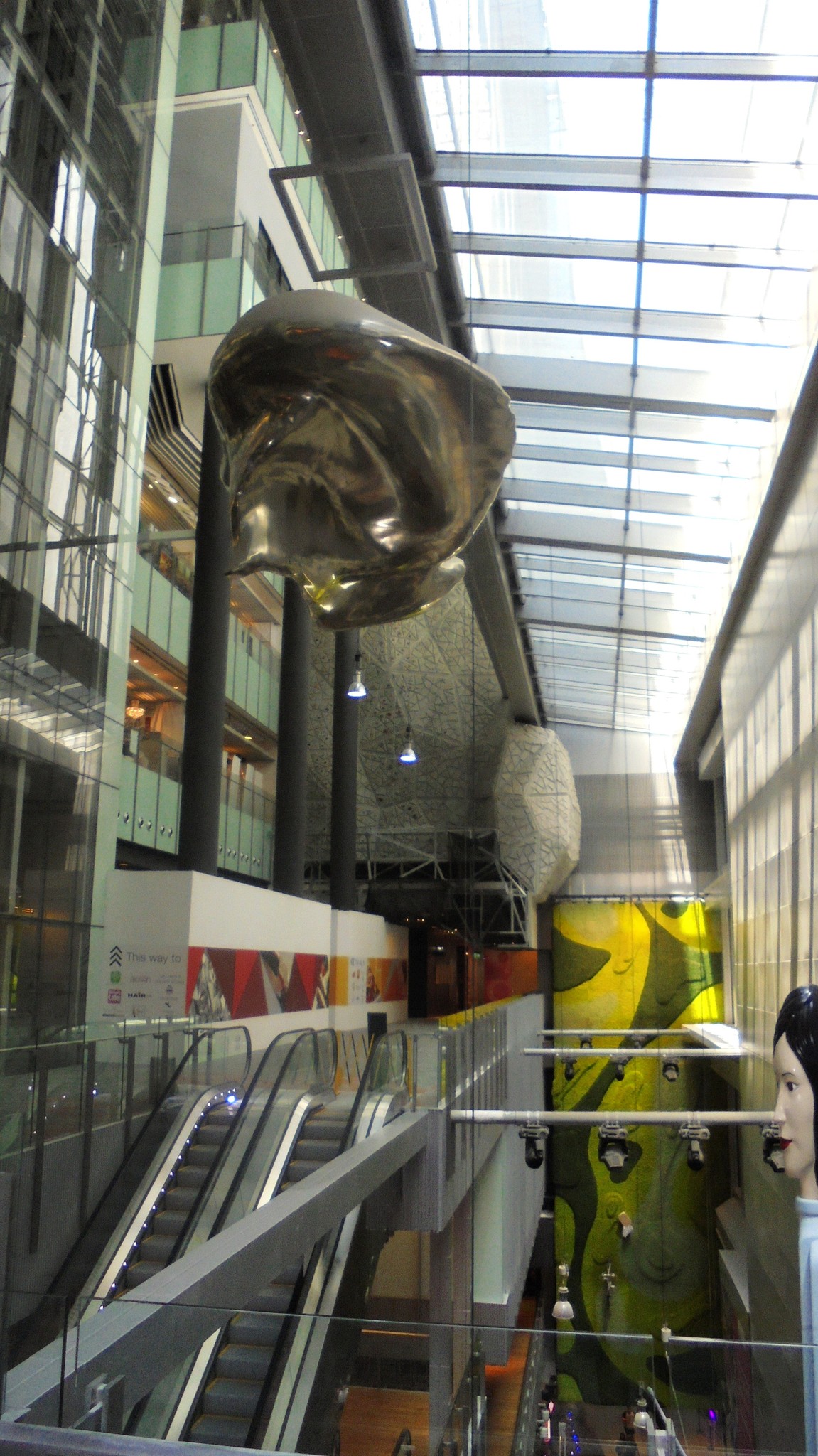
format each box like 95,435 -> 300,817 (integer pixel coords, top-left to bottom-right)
622,1405 -> 636,1438
615,1431 -> 640,1456
773,984 -> 818,1456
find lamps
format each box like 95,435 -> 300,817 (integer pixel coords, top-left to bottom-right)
551,1263 -> 576,1320
346,651 -> 366,698
126,699 -> 145,719
677,1122 -> 710,1174
613,1056 -> 629,1082
399,726 -> 416,763
633,1381 -> 649,1429
560,1056 -> 578,1081
516,1123 -> 548,1171
594,1124 -> 630,1171
579,1034 -> 593,1049
630,1034 -> 646,1049
661,1056 -> 681,1081
